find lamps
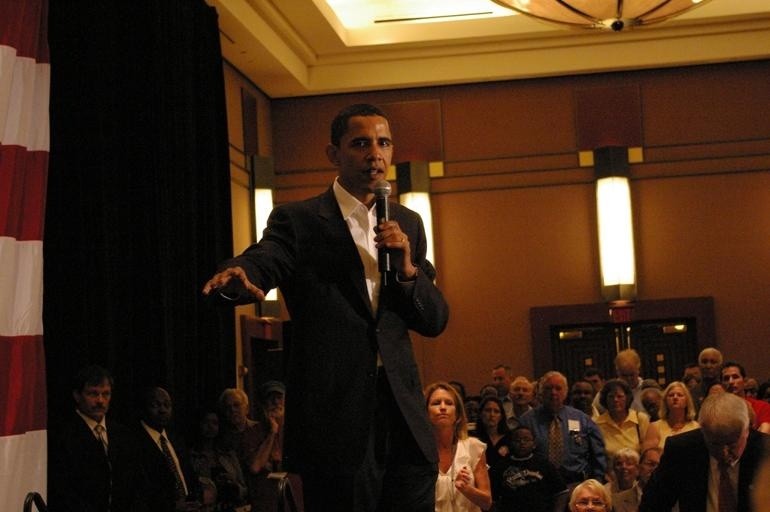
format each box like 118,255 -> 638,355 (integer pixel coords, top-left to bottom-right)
396,161 -> 436,287
492,0 -> 713,32
252,155 -> 283,316
592,145 -> 639,322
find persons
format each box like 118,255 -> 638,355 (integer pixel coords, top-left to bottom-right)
49,367 -> 285,510
427,349 -> 770,512
205,103 -> 450,510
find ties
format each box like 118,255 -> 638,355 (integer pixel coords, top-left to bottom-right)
94,425 -> 106,444
160,435 -> 185,496
717,459 -> 737,512
547,413 -> 562,464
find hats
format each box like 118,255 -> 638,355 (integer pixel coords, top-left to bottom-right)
260,380 -> 286,398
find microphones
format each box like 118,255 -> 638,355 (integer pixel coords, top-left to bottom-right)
373,180 -> 392,272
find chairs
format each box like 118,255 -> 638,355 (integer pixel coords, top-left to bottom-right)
23,492 -> 47,512
268,472 -> 298,512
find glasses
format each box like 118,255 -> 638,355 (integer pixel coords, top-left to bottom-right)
744,388 -> 758,393
